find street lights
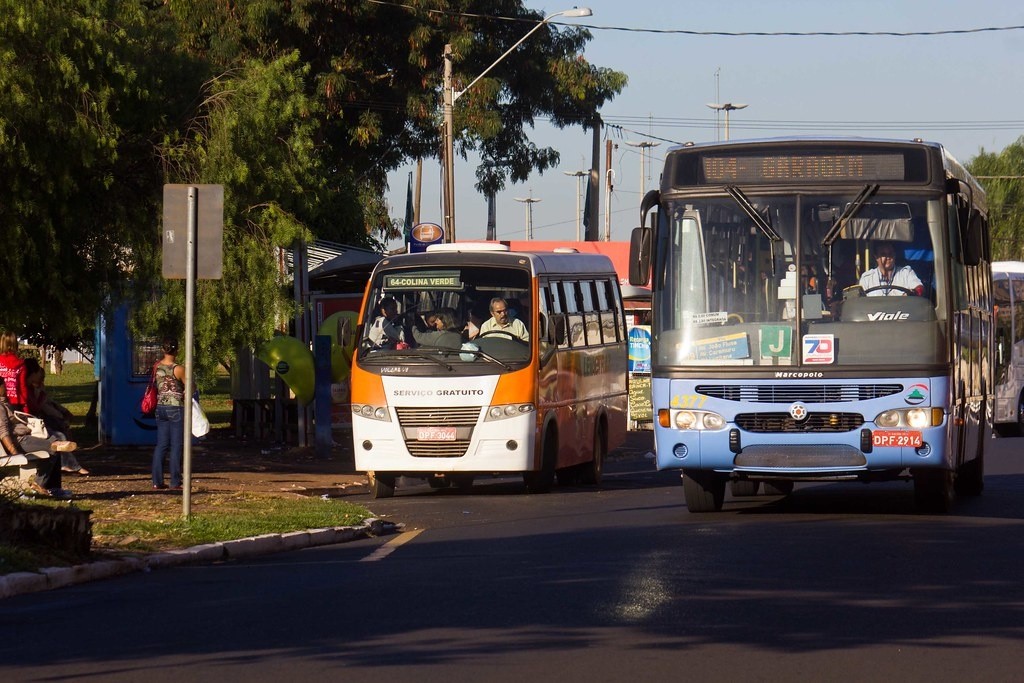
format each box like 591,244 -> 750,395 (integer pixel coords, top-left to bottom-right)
708,102 -> 747,140
563,172 -> 591,242
443,6 -> 593,243
626,142 -> 660,201
514,198 -> 540,240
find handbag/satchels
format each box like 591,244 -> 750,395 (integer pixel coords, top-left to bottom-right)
13,410 -> 48,440
191,397 -> 210,438
140,360 -> 160,415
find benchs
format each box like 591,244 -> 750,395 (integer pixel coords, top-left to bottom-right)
0,442 -> 50,486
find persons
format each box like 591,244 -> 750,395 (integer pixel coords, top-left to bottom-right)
0,330 -> 90,497
152,337 -> 197,490
859,240 -> 925,297
412,307 -> 468,350
369,297 -> 404,347
479,297 -> 529,342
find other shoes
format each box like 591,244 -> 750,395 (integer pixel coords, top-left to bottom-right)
48,488 -> 73,498
153,484 -> 183,490
50,440 -> 77,452
61,467 -> 95,477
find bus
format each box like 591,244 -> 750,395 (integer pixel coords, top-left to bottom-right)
628,135 -> 994,513
338,241 -> 628,497
992,261 -> 1024,425
